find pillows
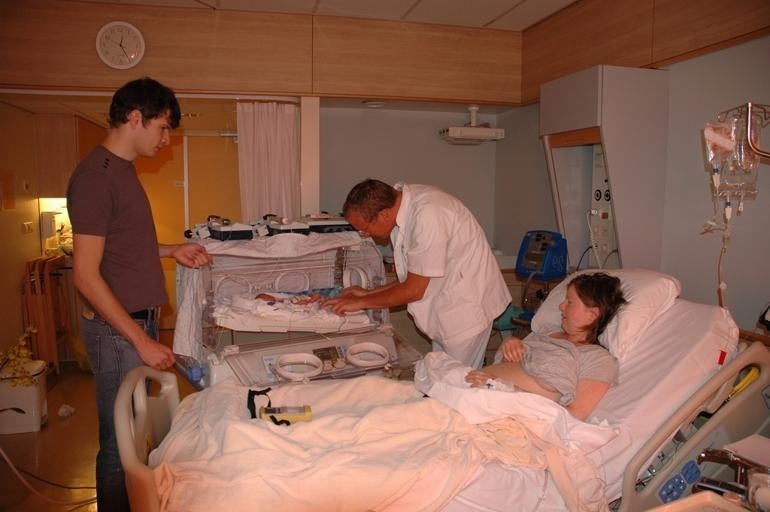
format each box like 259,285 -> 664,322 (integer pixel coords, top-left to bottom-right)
530,269 -> 681,361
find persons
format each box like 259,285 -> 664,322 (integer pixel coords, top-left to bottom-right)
318,178 -> 514,371
66,77 -> 214,511
254,291 -> 321,305
464,272 -> 627,421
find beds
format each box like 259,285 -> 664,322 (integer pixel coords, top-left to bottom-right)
113,270 -> 770,510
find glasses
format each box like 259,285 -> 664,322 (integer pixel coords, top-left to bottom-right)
358,216 -> 372,239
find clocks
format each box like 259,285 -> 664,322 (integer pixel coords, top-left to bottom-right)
96,21 -> 145,70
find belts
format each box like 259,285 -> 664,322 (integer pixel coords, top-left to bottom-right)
129,305 -> 161,320
